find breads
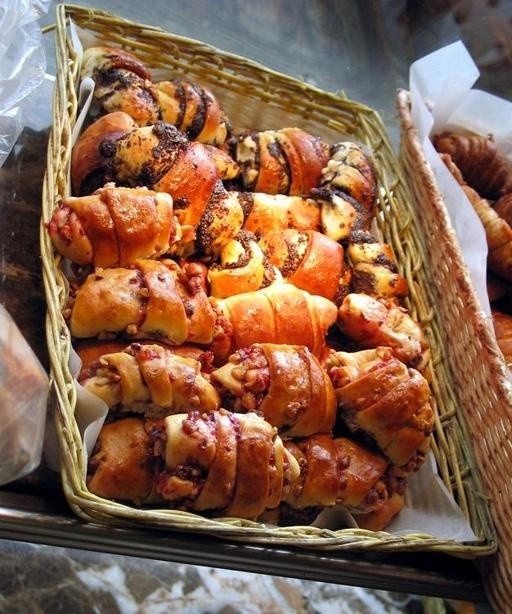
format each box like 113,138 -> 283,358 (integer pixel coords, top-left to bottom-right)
432,129 -> 512,377
46,47 -> 437,530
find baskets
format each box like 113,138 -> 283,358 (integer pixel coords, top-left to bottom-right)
397,87 -> 511,614
39,1 -> 498,560
